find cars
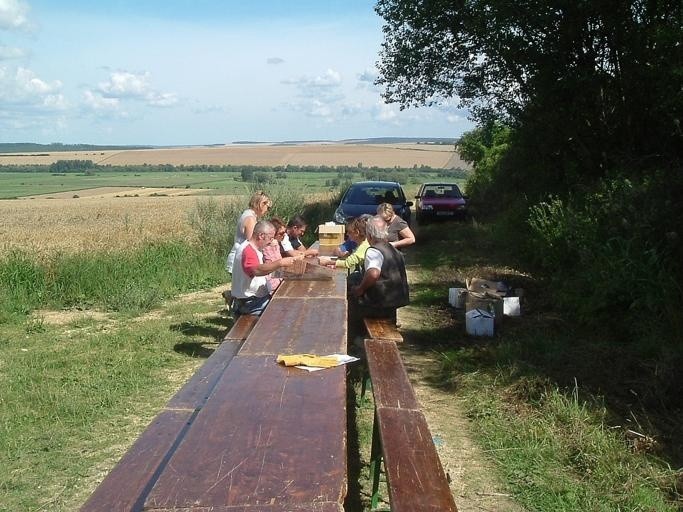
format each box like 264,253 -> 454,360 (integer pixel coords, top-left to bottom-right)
416,184 -> 469,224
334,181 -> 413,236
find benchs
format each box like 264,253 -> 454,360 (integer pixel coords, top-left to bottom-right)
81,314 -> 258,512
361,316 -> 456,512
146,268 -> 349,512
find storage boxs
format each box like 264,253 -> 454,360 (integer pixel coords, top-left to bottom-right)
313,223 -> 347,247
448,278 -> 526,338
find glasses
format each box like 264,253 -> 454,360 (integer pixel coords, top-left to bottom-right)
263,202 -> 272,212
384,215 -> 393,223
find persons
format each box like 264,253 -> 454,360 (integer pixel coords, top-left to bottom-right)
219,188 -> 416,345
